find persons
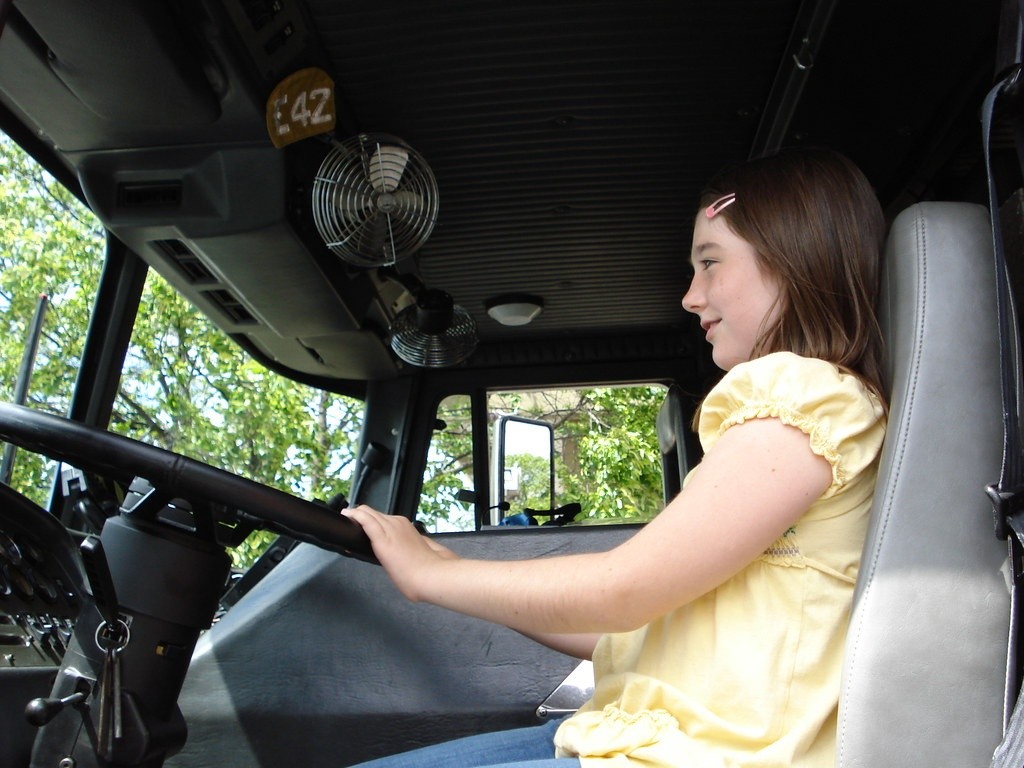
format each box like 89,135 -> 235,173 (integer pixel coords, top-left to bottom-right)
337,147 -> 894,767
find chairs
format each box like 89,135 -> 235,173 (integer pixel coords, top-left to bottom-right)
657,383 -> 704,506
836,201 -> 1015,767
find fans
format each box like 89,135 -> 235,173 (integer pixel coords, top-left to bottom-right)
311,130 -> 439,268
377,265 -> 479,369
